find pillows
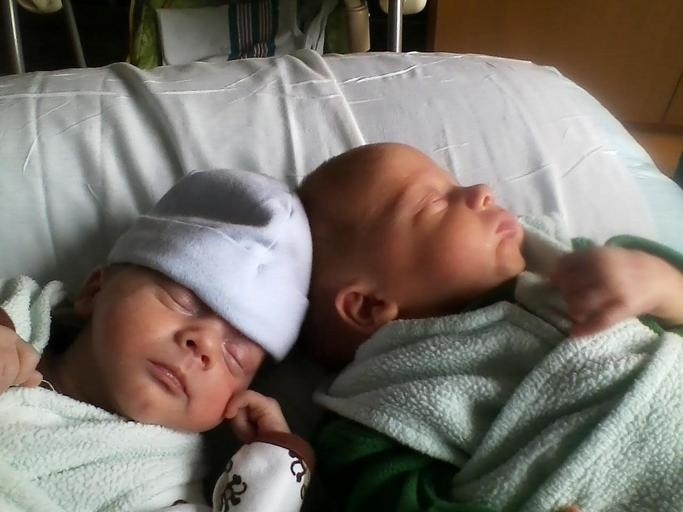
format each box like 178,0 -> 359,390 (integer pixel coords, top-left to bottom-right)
1,50 -> 682,293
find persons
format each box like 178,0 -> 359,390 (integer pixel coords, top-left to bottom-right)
295,140 -> 683,512
0,166 -> 316,512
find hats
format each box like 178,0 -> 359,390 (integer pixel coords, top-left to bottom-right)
103,168 -> 312,366
